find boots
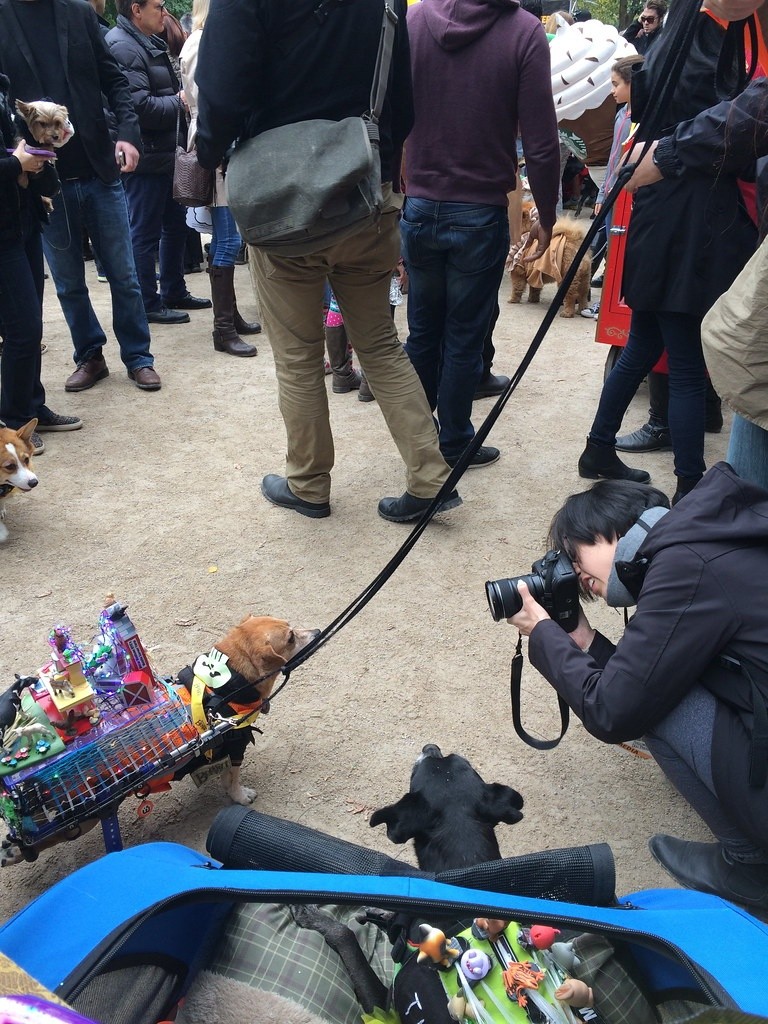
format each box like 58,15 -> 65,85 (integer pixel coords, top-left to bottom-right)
358,374 -> 378,402
324,325 -> 363,393
209,267 -> 262,357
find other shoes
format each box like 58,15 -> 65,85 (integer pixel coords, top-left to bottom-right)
672,477 -> 712,510
97,271 -> 109,282
581,300 -> 602,321
378,488 -> 465,521
141,302 -> 190,323
591,274 -> 604,288
578,438 -> 651,484
28,432 -> 45,455
33,405 -> 83,431
64,354 -> 109,392
469,372 -> 511,401
162,294 -> 213,309
324,360 -> 334,373
127,366 -> 162,389
704,421 -> 724,433
41,343 -> 48,355
440,444 -> 501,470
261,473 -> 331,517
613,417 -> 673,453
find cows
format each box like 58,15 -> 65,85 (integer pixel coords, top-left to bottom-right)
0,673 -> 39,748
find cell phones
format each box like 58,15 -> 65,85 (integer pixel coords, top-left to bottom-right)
119,151 -> 125,167
6,148 -> 56,158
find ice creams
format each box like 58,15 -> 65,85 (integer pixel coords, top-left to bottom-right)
547,19 -> 642,169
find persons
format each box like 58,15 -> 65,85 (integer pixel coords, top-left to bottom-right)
506,461 -> 768,924
194,0 -> 464,523
0,0 -> 161,392
0,0 -> 768,506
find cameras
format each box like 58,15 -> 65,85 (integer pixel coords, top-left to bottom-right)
483,548 -> 580,632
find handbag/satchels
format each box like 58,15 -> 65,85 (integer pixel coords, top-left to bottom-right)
227,117 -> 381,258
172,144 -> 214,207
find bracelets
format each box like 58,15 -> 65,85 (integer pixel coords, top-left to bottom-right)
653,148 -> 669,179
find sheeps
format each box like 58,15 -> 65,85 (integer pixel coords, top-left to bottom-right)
43,670 -> 77,698
12,723 -> 56,746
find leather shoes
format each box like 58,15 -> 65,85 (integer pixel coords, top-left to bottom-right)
650,832 -> 768,914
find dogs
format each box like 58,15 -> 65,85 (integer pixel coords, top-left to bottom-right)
0,611 -> 323,866
289,744 -> 585,1024
0,418 -> 41,543
508,201 -> 594,318
9,98 -> 70,215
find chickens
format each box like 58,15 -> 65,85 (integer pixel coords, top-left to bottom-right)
87,706 -> 102,723
49,707 -> 76,734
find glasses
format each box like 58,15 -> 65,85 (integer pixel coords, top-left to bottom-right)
641,16 -> 660,23
155,1 -> 166,16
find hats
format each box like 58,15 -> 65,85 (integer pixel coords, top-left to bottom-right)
574,9 -> 592,22
